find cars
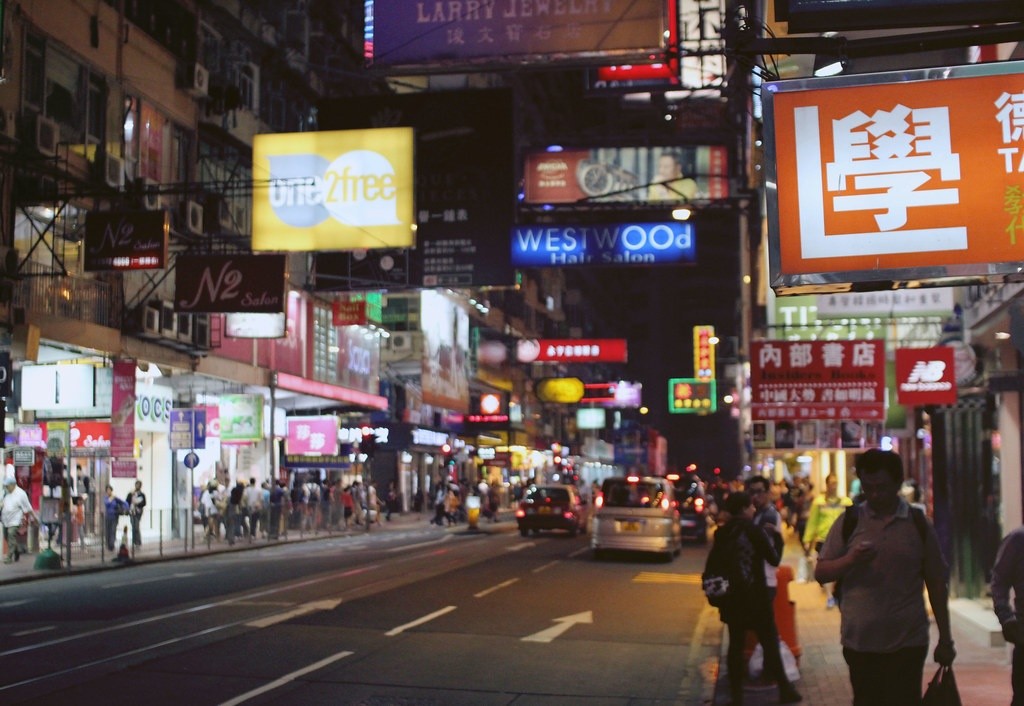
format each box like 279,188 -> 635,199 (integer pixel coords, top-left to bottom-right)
515,484 -> 587,538
587,476 -> 684,560
670,473 -> 710,542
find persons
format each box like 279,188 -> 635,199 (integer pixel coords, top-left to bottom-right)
991,528 -> 1024,706
814,449 -> 956,706
701,476 -> 803,706
579,479 -> 601,504
196,477 -> 425,546
126,481 -> 146,547
902,478 -> 936,623
104,485 -> 126,552
707,476 -> 813,563
49,464 -> 90,554
0,476 -> 39,564
429,476 -> 537,528
848,467 -> 865,500
803,475 -> 854,609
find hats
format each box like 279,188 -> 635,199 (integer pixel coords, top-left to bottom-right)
3,476 -> 16,486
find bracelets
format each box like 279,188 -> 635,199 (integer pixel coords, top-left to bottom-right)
940,638 -> 954,647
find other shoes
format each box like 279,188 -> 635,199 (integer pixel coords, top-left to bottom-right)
780,689 -> 802,705
4,559 -> 12,564
14,551 -> 19,561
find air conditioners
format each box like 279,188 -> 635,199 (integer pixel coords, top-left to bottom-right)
95,151 -> 125,188
390,331 -> 410,350
18,113 -> 59,157
134,297 -> 193,342
179,199 -> 203,236
182,61 -> 210,97
132,177 -> 161,210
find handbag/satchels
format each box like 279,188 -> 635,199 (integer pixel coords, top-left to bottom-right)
921,660 -> 961,706
116,498 -> 123,514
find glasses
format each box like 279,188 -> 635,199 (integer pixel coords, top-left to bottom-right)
747,488 -> 767,495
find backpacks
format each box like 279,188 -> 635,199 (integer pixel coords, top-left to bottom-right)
307,483 -> 318,507
703,521 -> 749,607
